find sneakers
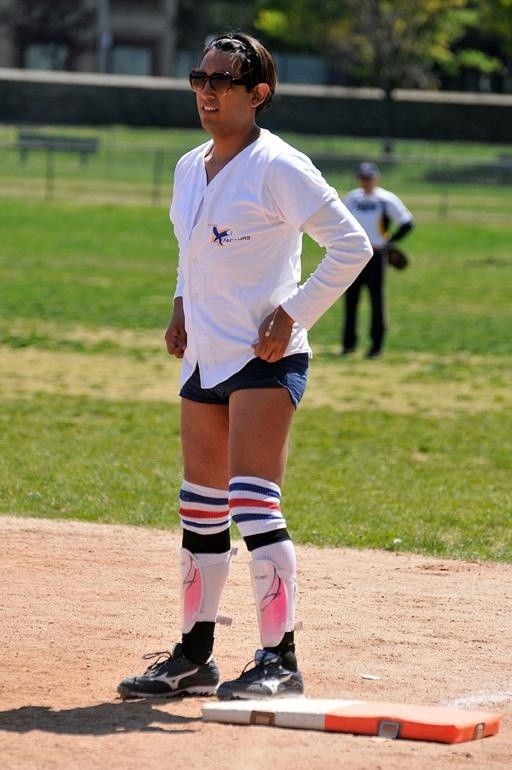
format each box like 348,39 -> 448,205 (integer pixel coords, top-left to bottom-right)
219,650 -> 303,700
117,643 -> 221,697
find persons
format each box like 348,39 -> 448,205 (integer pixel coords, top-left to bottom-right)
339,158 -> 415,359
116,26 -> 380,703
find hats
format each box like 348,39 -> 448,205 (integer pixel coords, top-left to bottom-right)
358,162 -> 381,177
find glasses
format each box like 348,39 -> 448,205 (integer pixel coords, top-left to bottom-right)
188,69 -> 248,92
